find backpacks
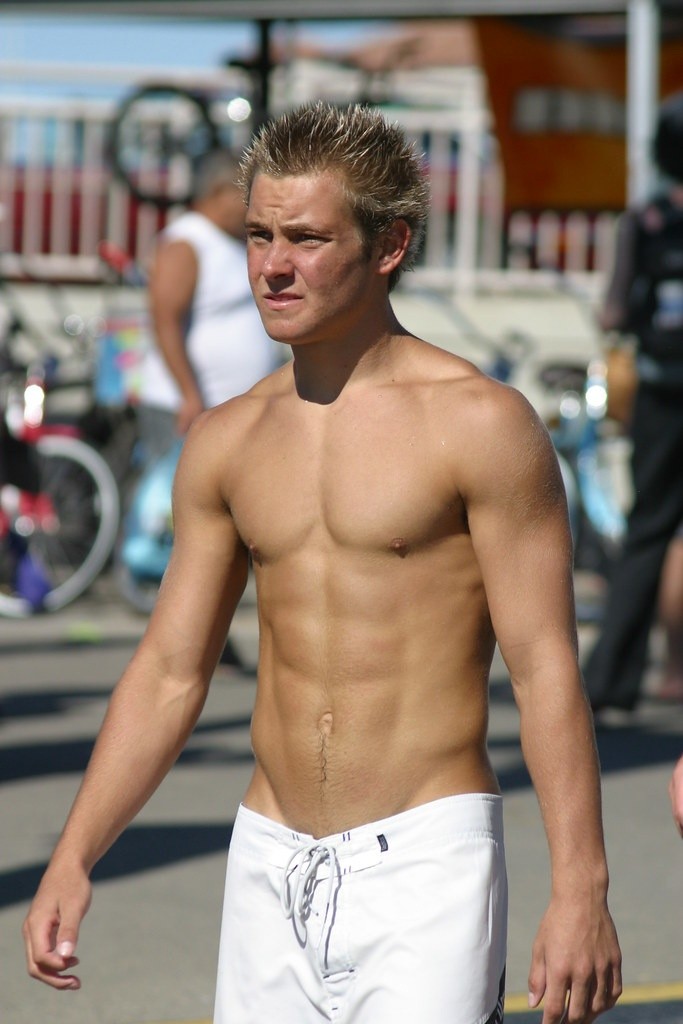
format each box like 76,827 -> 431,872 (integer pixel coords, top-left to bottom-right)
639,194 -> 683,356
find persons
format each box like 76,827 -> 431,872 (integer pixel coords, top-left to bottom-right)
580,100 -> 683,838
0,369 -> 52,612
19,99 -> 628,1024
113,141 -> 295,681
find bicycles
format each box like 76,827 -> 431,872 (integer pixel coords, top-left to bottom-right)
0,431 -> 123,620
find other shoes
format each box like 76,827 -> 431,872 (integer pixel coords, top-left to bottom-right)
592,705 -> 629,730
117,568 -> 159,617
